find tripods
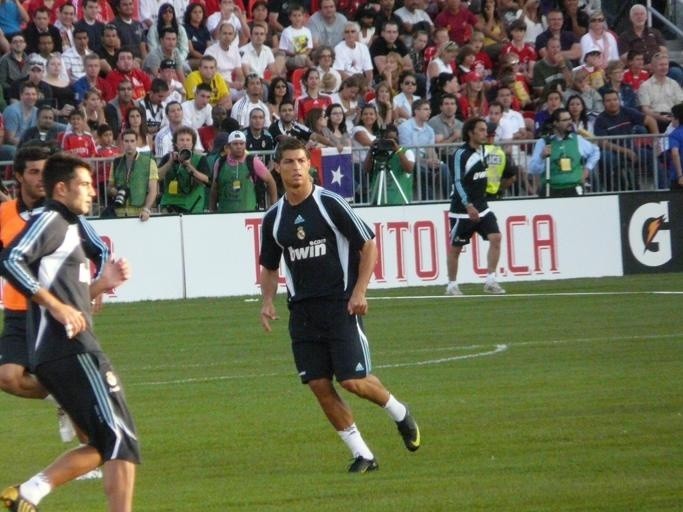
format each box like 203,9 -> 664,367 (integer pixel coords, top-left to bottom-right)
368,165 -> 413,204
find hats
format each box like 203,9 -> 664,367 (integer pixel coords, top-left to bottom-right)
466,72 -> 483,81
580,46 -> 600,62
160,59 -> 176,69
228,130 -> 246,144
29,57 -> 44,72
471,31 -> 486,40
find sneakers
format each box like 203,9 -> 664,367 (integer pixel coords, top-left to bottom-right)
76,468 -> 104,479
445,286 -> 463,296
56,403 -> 76,443
1,485 -> 39,512
395,408 -> 421,453
346,450 -> 378,473
484,279 -> 506,294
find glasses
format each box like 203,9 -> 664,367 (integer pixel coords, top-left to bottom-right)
162,9 -> 173,13
590,18 -> 605,24
103,25 -> 118,31
320,55 -> 332,58
30,62 -> 44,66
246,74 -> 259,80
404,81 -> 417,86
444,42 -> 455,51
506,60 -> 520,66
345,31 -> 356,34
275,84 -> 286,88
118,87 -> 133,91
160,61 -> 176,66
560,117 -> 572,122
331,112 -> 343,115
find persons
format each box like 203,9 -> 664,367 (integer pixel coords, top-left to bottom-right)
443,115 -> 506,299
257,136 -> 421,472
666,103 -> 683,273
1,1 -> 682,221
1,153 -> 143,511
0,145 -> 77,442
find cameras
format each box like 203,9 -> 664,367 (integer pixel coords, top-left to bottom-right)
114,185 -> 128,208
179,148 -> 193,163
372,131 -> 399,171
